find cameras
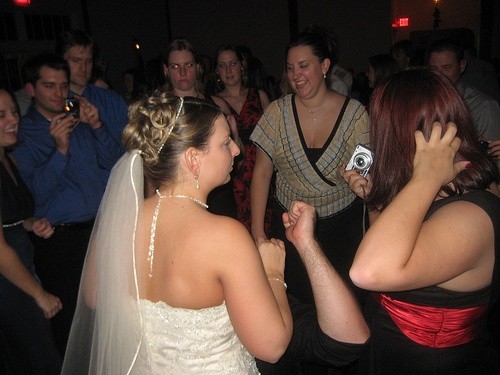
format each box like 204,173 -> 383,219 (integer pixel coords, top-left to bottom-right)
345,143 -> 374,178
64,98 -> 80,122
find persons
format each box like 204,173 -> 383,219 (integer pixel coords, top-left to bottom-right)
6,54 -> 121,359
255,201 -> 371,375
279,28 -> 500,168
348,68 -> 500,375
249,22 -> 373,308
56,28 -> 276,242
60,92 -> 294,375
0,85 -> 63,375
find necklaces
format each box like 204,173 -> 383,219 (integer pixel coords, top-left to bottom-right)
147,190 -> 208,277
301,101 -> 319,121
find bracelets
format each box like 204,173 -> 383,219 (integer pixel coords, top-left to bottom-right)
268,278 -> 287,290
